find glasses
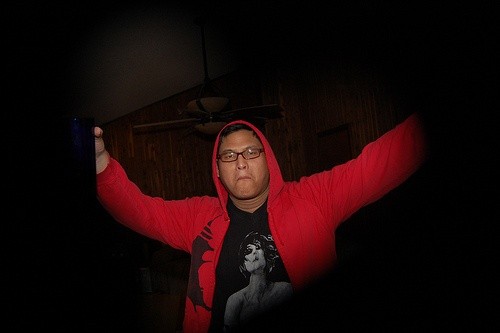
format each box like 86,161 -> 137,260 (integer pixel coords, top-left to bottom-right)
217,147 -> 264,162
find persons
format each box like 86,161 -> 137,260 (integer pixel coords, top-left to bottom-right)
222,231 -> 293,333
94,112 -> 431,333
